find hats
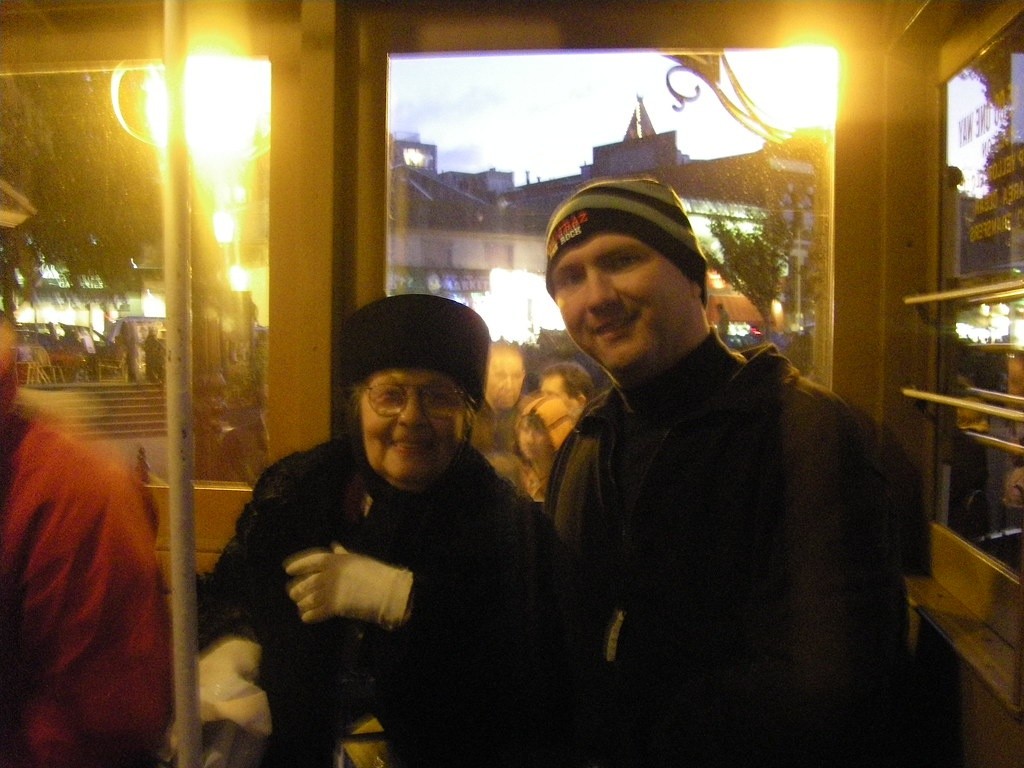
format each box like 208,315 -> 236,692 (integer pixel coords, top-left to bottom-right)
545,178 -> 709,307
338,294 -> 488,411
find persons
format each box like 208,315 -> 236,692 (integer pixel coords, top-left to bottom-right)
0,320 -> 174,768
544,174 -> 910,768
466,342 -> 598,504
167,296 -> 565,768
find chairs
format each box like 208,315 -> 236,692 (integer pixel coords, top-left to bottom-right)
12,343 -> 130,385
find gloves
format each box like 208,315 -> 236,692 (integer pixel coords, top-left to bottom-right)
159,635 -> 272,762
283,540 -> 413,628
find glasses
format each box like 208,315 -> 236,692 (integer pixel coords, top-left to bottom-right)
353,382 -> 472,417
